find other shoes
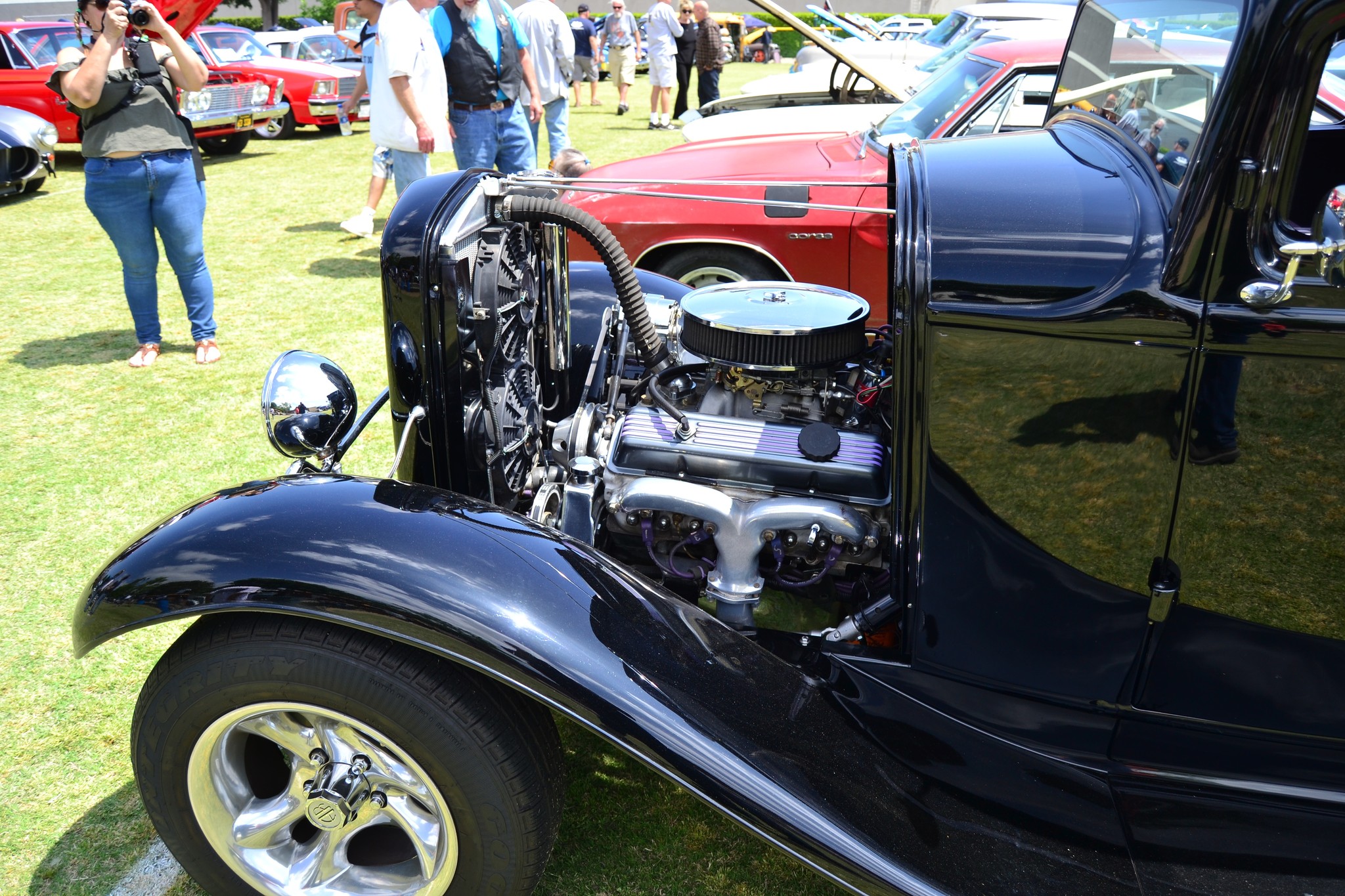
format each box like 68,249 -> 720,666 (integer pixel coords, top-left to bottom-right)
340,215 -> 373,238
617,104 -> 626,115
625,105 -> 629,111
660,122 -> 681,131
648,122 -> 662,130
590,99 -> 603,105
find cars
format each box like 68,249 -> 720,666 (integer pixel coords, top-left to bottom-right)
0,104 -> 58,198
0,0 -> 290,164
560,38 -> 1345,346
69,2 -> 1345,895
583,0 -> 1344,144
184,16 -> 370,141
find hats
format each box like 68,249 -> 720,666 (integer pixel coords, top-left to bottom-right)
374,0 -> 386,5
1176,137 -> 1189,148
578,3 -> 590,13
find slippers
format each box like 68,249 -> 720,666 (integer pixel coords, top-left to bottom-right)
128,343 -> 159,368
196,339 -> 221,364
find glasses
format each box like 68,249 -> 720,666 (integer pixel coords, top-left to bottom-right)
547,159 -> 566,178
1154,126 -> 1162,132
1132,97 -> 1138,101
682,9 -> 693,13
613,6 -> 623,9
88,0 -> 131,11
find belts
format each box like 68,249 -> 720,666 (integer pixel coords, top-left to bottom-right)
454,95 -> 519,111
608,44 -> 631,50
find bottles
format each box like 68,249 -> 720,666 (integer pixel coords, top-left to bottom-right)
337,104 -> 352,137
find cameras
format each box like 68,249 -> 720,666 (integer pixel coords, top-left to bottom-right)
118,0 -> 149,26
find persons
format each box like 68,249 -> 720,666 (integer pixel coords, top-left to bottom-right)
673,0 -> 697,121
1155,137 -> 1191,187
337,0 -> 396,237
599,0 -> 641,115
510,0 -> 592,208
691,1 -> 725,117
1095,93 -> 1119,126
1131,117 -> 1167,165
1337,197 -> 1345,222
1116,89 -> 1147,139
56,0 -> 224,364
548,148 -> 592,202
298,402 -> 306,414
270,409 -> 274,420
647,0 -> 685,131
569,3 -> 602,106
426,0 -> 542,174
372,0 -> 448,197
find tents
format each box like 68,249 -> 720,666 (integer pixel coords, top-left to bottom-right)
738,14 -> 773,63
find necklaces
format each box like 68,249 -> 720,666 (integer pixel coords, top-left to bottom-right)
90,35 -> 97,45
